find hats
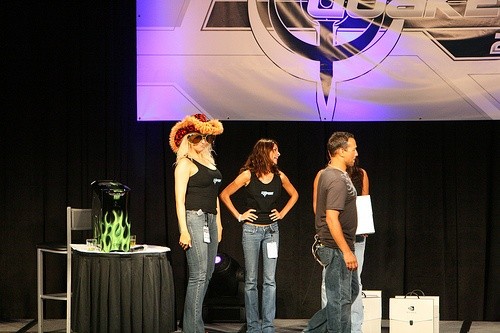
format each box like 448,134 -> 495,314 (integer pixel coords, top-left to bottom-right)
169,114 -> 224,153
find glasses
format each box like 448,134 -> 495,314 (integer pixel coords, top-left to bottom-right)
188,135 -> 216,144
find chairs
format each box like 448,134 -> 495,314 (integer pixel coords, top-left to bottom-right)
35,201 -> 92,333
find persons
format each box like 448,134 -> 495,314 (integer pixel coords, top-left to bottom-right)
301,131 -> 369,333
219,139 -> 298,333
169,114 -> 223,333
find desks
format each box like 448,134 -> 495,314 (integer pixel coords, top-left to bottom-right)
70,244 -> 175,333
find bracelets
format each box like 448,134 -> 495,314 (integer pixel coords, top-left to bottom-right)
238,213 -> 242,223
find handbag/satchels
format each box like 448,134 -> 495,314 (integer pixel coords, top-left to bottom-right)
389,290 -> 439,333
361,290 -> 382,333
